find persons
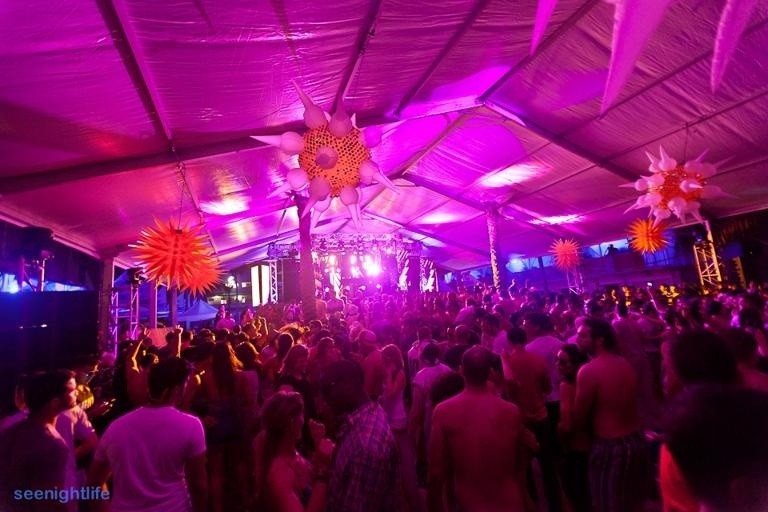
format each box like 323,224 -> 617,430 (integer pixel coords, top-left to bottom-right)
1,263 -> 766,511
